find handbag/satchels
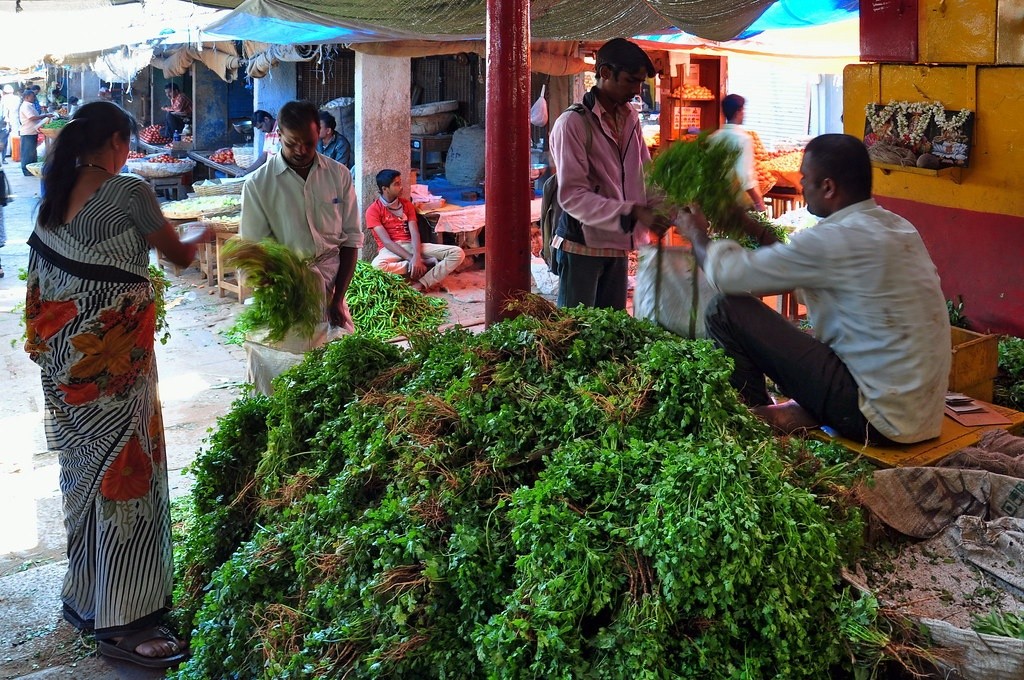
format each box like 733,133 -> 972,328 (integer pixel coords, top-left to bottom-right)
632,224 -> 721,341
530,86 -> 549,128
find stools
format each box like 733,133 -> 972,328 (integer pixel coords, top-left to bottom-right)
809,391 -> 1024,469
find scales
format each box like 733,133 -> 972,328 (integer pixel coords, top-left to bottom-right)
233,121 -> 253,148
531,163 -> 549,199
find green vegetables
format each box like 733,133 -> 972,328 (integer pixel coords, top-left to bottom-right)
41,119 -> 67,129
946,294 -> 1024,413
147,264 -> 175,346
646,131 -> 789,249
222,236 -> 327,344
170,289 -> 935,680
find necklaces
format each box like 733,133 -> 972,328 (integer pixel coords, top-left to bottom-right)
76,163 -> 106,173
288,160 -> 313,170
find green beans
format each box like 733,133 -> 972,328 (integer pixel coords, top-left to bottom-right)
344,260 -> 449,340
966,608 -> 1024,639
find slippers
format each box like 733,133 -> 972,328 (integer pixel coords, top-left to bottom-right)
99,622 -> 188,666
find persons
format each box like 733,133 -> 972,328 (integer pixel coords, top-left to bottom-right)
704,94 -> 765,211
365,169 -> 465,292
0,85 -> 113,176
239,102 -> 364,354
236,110 -> 281,178
25,102 -> 216,668
317,111 -> 350,167
549,38 -> 673,311
0,165 -> 9,278
159,82 -> 192,138
674,134 -> 951,447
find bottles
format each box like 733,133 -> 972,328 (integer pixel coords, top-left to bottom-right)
173,130 -> 181,142
182,124 -> 192,136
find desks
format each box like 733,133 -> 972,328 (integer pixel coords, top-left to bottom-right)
149,151 -> 543,306
765,193 -> 807,219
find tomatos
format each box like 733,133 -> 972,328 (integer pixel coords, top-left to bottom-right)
209,149 -> 234,165
128,126 -> 193,163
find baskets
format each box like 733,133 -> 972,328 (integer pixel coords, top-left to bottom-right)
232,145 -> 254,169
130,165 -> 189,177
198,214 -> 239,233
409,101 -> 458,134
192,181 -> 245,196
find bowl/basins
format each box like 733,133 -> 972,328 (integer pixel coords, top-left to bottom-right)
233,120 -> 252,133
531,163 -> 549,180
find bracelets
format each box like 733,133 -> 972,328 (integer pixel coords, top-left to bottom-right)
755,200 -> 762,205
244,170 -> 248,174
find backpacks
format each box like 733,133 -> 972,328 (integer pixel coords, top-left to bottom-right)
542,102 -> 592,275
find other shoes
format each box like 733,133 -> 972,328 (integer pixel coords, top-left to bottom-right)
438,283 -> 449,293
164,142 -> 173,148
